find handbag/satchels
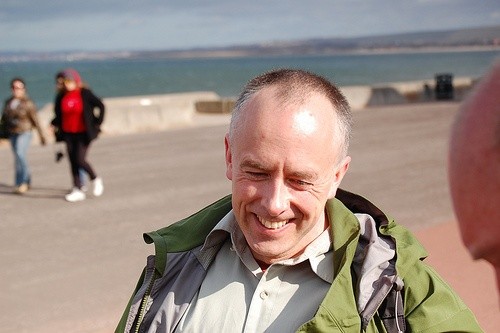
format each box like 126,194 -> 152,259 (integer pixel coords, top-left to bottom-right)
0,114 -> 13,138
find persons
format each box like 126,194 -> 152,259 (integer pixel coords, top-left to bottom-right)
114,67 -> 486,333
0,67 -> 106,202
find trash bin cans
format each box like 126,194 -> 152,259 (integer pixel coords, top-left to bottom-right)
435,74 -> 453,100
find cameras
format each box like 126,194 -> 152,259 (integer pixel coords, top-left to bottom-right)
55,152 -> 63,161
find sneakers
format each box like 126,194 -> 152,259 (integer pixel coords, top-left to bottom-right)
91,178 -> 103,197
64,189 -> 85,202
13,182 -> 30,194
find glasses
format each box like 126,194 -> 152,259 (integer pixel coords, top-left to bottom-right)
11,86 -> 23,90
